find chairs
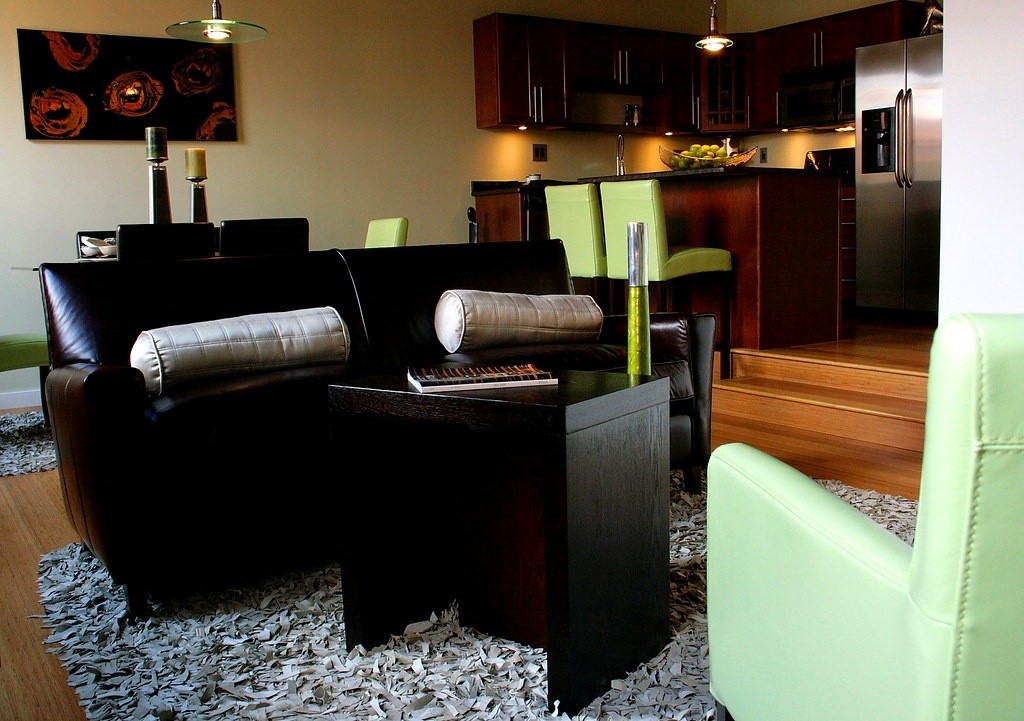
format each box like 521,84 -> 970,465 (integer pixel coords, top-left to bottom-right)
365,218 -> 408,248
75,217 -> 309,260
600,179 -> 733,382
545,183 -> 625,317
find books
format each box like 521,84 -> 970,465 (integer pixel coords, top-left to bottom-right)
408,363 -> 558,394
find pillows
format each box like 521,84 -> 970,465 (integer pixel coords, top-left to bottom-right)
434,289 -> 605,353
128,307 -> 352,394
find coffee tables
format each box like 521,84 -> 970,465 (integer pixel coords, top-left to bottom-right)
328,367 -> 674,715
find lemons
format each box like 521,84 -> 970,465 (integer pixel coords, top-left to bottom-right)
670,145 -> 741,170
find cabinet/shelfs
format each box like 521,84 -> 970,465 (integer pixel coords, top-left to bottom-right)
474,0 -> 943,137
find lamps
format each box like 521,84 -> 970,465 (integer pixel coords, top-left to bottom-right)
694,0 -> 733,51
166,0 -> 268,44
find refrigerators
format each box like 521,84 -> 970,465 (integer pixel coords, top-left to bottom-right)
854,33 -> 943,318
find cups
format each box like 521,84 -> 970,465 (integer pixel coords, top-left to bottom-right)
529,174 -> 541,180
526,176 -> 528,183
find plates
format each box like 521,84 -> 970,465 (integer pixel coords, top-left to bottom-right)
657,146 -> 758,170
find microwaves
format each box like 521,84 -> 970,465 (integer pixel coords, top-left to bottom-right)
781,77 -> 855,134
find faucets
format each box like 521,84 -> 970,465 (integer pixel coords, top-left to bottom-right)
615,134 -> 627,177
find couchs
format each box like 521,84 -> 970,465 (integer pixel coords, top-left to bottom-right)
40,238 -> 716,625
709,313 -> 1024,721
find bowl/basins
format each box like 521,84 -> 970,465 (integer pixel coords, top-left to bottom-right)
81,246 -> 99,257
99,246 -> 117,256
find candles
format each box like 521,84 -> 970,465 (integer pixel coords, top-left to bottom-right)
185,148 -> 206,177
145,127 -> 167,157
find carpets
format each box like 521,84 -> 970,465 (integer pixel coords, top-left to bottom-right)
36,478 -> 918,721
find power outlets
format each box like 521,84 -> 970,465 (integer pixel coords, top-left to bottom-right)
760,148 -> 767,163
533,144 -> 547,161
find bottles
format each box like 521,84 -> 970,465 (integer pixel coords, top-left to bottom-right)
624,104 -> 632,126
631,105 -> 641,126
